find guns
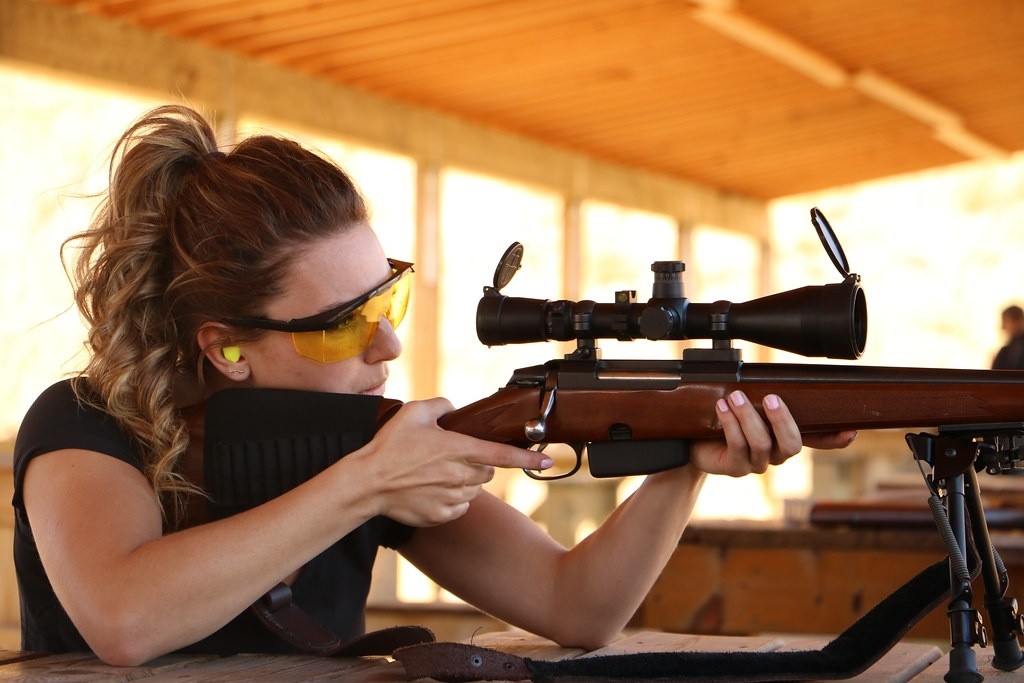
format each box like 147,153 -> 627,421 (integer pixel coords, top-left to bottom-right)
202,206 -> 1024,683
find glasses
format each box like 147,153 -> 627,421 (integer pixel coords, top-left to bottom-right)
225,258 -> 416,366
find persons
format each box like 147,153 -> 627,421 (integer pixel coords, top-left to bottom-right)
989,306 -> 1024,371
8,104 -> 858,668
